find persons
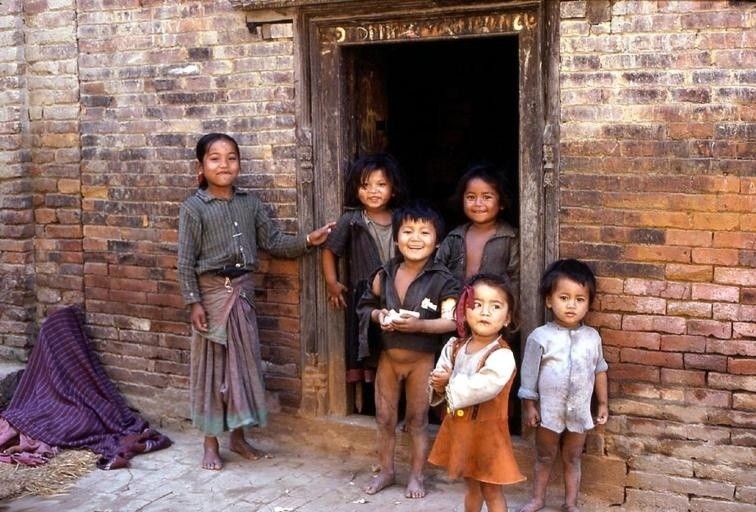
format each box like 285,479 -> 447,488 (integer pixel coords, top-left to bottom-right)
430,174 -> 519,337
430,270 -> 528,512
516,258 -> 608,512
177,133 -> 336,471
322,160 -> 401,411
356,208 -> 457,498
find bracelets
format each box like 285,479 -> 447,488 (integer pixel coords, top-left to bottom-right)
306,235 -> 311,247
